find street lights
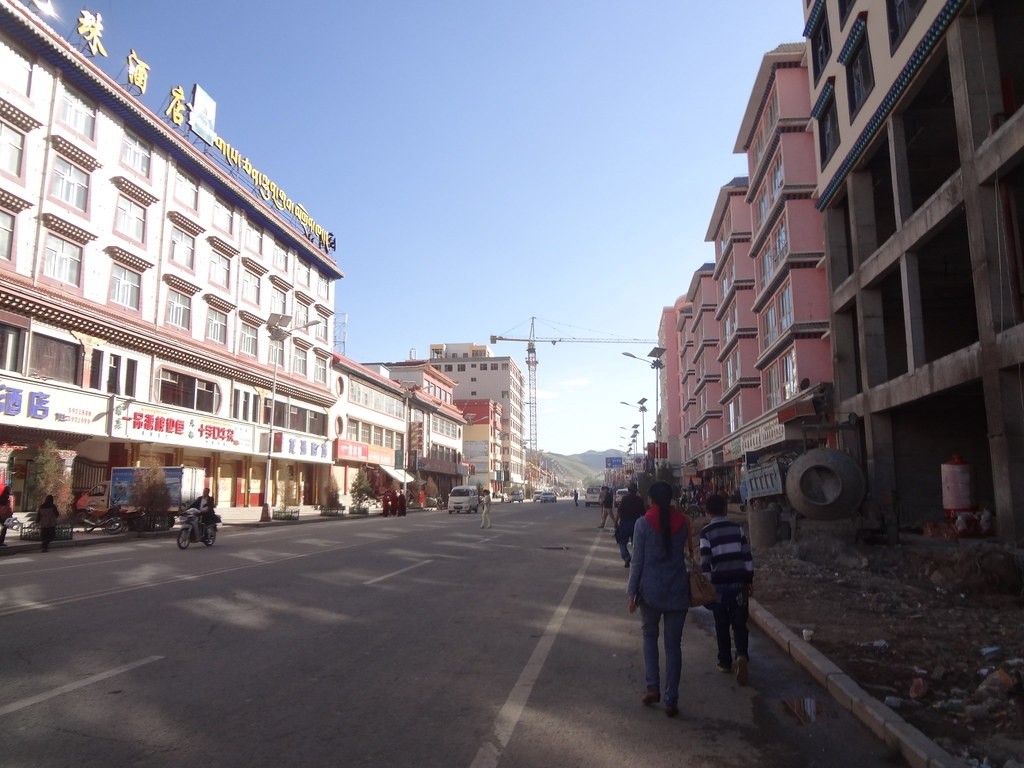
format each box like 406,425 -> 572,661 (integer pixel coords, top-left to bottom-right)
620,398 -> 648,473
621,347 -> 667,479
618,422 -> 639,475
403,384 -> 435,497
258,319 -> 323,523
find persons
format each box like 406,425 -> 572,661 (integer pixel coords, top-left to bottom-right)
480,490 -> 493,528
383,490 -> 406,517
597,487 -> 615,528
681,482 -> 713,510
0,485 -> 13,546
36,495 -> 59,552
698,495 -> 754,685
625,483 -> 691,716
574,490 -> 578,506
188,488 -> 215,540
614,484 -> 645,568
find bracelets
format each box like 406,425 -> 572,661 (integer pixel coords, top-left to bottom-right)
614,521 -> 617,522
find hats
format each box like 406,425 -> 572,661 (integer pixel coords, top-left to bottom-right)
628,482 -> 638,492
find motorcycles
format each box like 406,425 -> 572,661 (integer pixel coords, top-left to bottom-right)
176,508 -> 217,550
81,492 -> 125,535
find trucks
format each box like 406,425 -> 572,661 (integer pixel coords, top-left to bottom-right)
74,466 -> 206,530
738,460 -> 790,512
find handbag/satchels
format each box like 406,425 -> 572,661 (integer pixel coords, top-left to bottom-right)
680,513 -> 719,608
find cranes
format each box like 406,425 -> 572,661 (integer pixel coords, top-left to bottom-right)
490,316 -> 659,500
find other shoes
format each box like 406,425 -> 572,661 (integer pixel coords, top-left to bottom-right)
716,663 -> 730,673
735,655 -> 749,686
624,556 -> 631,568
41,547 -> 48,553
0,543 -> 8,546
642,692 -> 661,704
665,703 -> 678,717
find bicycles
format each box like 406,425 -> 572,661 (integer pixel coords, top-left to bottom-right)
678,487 -> 702,520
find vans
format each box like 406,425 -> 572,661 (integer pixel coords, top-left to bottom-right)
585,486 -> 602,507
447,485 -> 479,514
511,490 -> 524,503
533,490 -> 544,502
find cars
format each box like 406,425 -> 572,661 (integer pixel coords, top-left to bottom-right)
540,492 -> 557,503
614,489 -> 628,508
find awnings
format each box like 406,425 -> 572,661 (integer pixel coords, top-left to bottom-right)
381,465 -> 416,482
